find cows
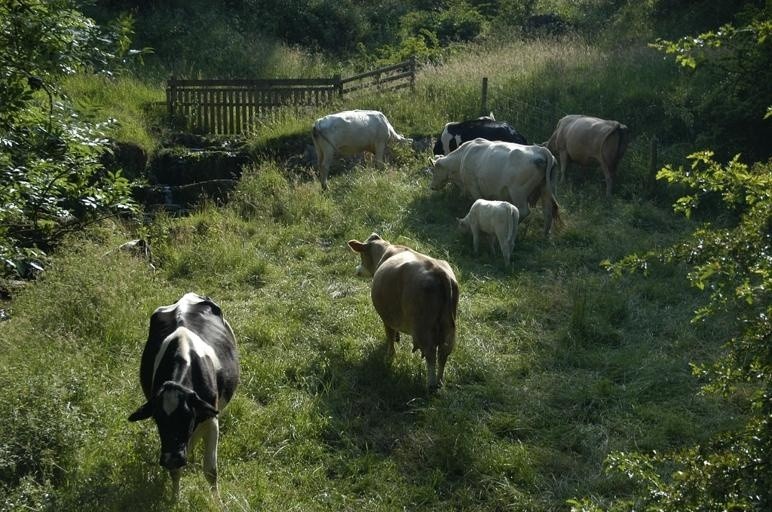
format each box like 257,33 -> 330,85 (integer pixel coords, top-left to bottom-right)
532,112 -> 630,201
425,136 -> 563,243
309,107 -> 415,194
429,112 -> 528,156
348,231 -> 462,397
125,290 -> 242,511
455,196 -> 524,267
